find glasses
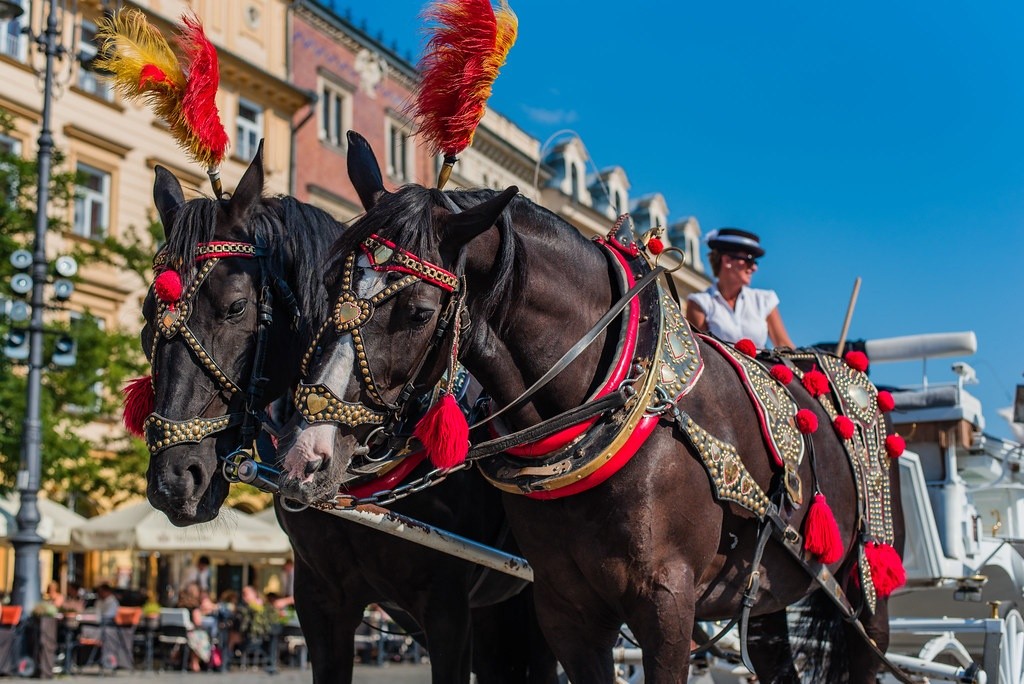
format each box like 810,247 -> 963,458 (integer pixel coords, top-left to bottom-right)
723,255 -> 758,269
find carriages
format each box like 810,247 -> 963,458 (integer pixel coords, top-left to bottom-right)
124,0 -> 1024,684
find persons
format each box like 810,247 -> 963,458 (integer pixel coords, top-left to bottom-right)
40,555 -> 429,673
685,227 -> 796,354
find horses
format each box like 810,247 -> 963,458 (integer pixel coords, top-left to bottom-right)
139,136 -> 804,684
276,130 -> 907,684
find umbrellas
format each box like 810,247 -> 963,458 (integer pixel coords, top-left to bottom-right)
0,485 -> 295,604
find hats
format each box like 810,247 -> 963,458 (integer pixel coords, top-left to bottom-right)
708,228 -> 764,259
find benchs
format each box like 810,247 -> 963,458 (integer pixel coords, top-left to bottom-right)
860,326 -> 988,561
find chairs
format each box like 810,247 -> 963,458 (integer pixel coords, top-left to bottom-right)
0,586 -> 421,679
